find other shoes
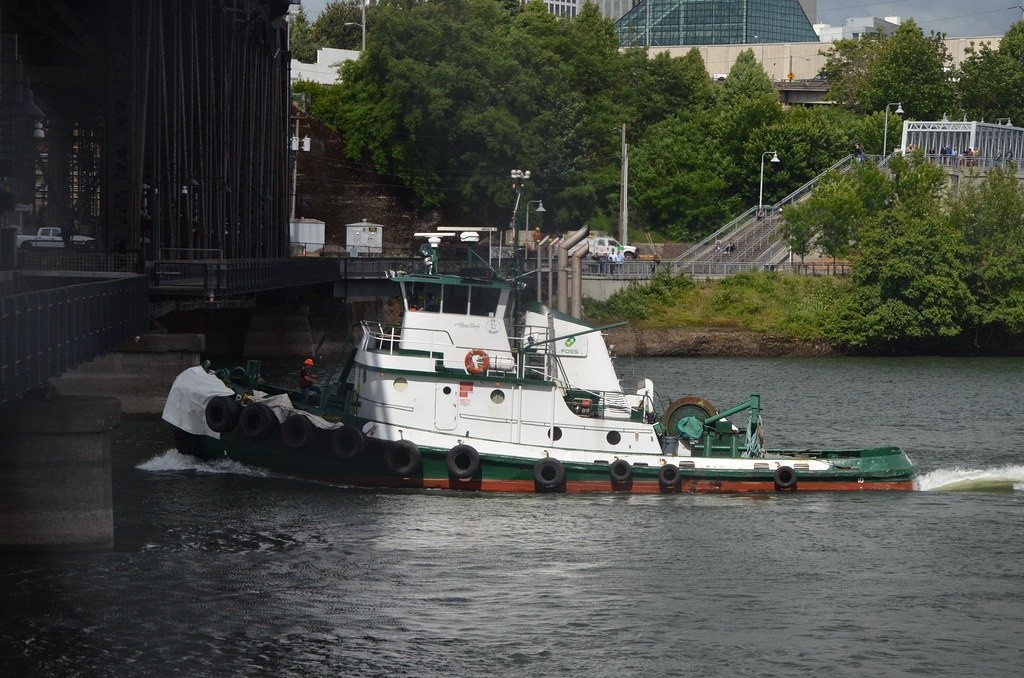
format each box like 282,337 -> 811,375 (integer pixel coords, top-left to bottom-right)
314,406 -> 319,408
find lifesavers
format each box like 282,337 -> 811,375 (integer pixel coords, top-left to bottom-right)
658,463 -> 683,487
533,457 -> 567,490
279,414 -> 315,452
383,439 -> 421,477
238,402 -> 274,442
328,424 -> 364,462
774,464 -> 798,488
205,394 -> 238,433
465,347 -> 490,375
609,458 -> 632,483
445,444 -> 480,480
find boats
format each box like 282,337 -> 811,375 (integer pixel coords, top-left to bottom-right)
172,240 -> 921,495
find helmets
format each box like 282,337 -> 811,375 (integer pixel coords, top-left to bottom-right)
536,239 -> 540,242
304,358 -> 314,365
536,227 -> 539,230
549,239 -> 553,242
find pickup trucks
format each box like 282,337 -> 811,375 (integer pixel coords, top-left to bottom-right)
17,226 -> 95,250
568,238 -> 639,260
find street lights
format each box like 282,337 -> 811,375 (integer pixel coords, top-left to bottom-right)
346,23 -> 366,54
524,200 -> 547,270
788,56 -> 810,81
759,151 -> 780,211
883,102 -> 904,160
754,35 -> 764,71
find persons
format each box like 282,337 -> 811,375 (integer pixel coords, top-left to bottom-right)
893,143 -> 1013,169
299,359 -> 322,403
756,207 -> 764,219
716,241 -> 736,256
650,255 -> 660,274
523,336 -> 539,353
417,292 -> 437,310
533,227 -> 541,249
854,140 -> 865,165
593,249 -> 625,273
